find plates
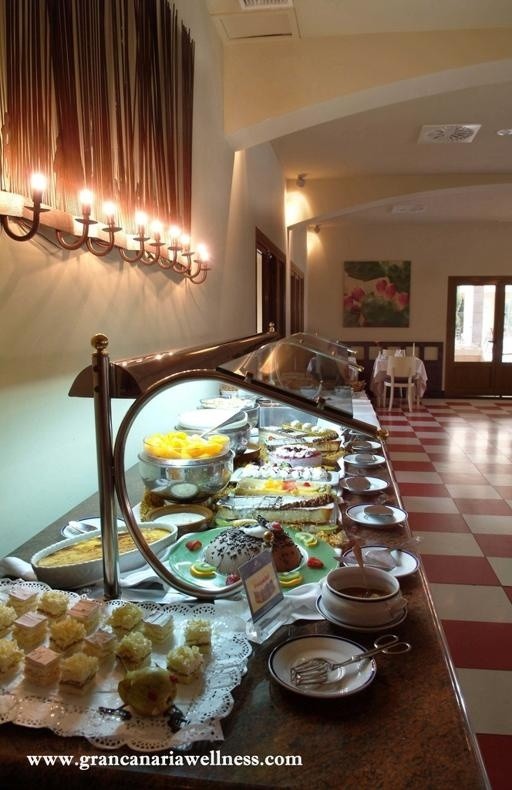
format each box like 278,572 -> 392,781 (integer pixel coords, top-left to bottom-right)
268,633 -> 380,700
338,474 -> 391,497
338,547 -> 421,579
342,455 -> 386,469
58,515 -> 128,541
166,523 -> 341,594
316,594 -> 409,633
352,440 -> 382,451
230,470 -> 340,487
344,502 -> 409,530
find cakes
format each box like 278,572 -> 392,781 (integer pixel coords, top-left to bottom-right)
24,642 -> 58,687
114,631 -> 153,670
49,616 -> 86,650
221,495 -> 333,526
167,643 -> 200,681
84,626 -> 117,663
142,609 -> 174,643
165,514 -> 338,597
0,639 -> 22,681
271,444 -> 322,467
58,651 -> 98,696
1,606 -> 16,635
37,589 -> 68,616
13,611 -> 47,648
8,586 -> 38,614
67,597 -> 101,634
183,620 -> 214,649
36,528 -> 172,567
232,477 -> 333,496
239,464 -> 331,480
105,600 -> 140,632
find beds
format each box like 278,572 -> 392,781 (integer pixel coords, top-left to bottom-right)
0,363 -> 497,788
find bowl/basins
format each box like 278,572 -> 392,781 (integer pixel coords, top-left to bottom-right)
323,565 -> 401,628
227,498 -> 335,524
202,402 -> 260,430
176,424 -> 251,469
134,446 -> 238,510
29,522 -> 180,590
145,502 -> 214,536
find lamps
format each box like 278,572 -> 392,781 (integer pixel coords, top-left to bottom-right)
137,219 -> 165,265
157,225 -> 183,269
119,210 -> 150,262
87,199 -> 122,256
56,189 -> 98,250
315,224 -> 321,233
3,173 -> 50,242
187,252 -> 210,284
173,233 -> 195,273
182,242 -> 207,279
296,174 -> 306,186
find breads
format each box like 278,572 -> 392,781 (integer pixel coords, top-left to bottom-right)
301,428 -> 342,452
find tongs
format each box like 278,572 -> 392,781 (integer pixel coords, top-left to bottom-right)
289,634 -> 411,688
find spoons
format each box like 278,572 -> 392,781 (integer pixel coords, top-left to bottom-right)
67,519 -> 97,534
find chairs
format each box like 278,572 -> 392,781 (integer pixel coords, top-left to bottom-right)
382,349 -> 405,398
384,356 -> 417,412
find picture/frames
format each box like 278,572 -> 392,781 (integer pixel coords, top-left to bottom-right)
342,261 -> 410,328
